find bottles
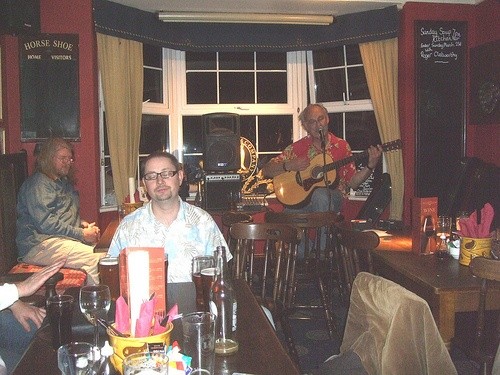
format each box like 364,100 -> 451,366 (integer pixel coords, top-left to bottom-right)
208,246 -> 239,354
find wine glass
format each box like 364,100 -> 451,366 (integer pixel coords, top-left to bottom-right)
436,216 -> 452,259
79,283 -> 112,346
423,217 -> 436,255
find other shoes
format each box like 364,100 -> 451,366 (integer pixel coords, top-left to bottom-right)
312,252 -> 327,261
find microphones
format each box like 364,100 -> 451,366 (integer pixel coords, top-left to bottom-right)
318,125 -> 325,146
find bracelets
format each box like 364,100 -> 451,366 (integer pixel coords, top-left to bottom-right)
366,164 -> 373,171
283,161 -> 287,173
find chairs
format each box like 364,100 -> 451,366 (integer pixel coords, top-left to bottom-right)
221,211 -> 500,375
0,149 -> 86,305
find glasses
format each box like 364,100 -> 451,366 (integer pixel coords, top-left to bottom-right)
54,156 -> 73,163
142,170 -> 178,180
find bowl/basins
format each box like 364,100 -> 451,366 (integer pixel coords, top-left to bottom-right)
449,247 -> 460,259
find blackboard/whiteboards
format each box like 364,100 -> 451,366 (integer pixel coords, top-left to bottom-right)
17,32 -> 82,142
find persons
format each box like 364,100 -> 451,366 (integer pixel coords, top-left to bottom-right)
261,102 -> 383,261
0,256 -> 67,375
16,135 -> 108,287
106,151 -> 276,332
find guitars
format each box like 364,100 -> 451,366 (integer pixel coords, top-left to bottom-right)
272,139 -> 403,210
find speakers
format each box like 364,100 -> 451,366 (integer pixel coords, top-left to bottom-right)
0,150 -> 28,275
204,174 -> 242,209
201,112 -> 241,173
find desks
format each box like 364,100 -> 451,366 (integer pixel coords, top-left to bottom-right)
10,280 -> 298,375
93,216 -> 120,253
341,218 -> 500,361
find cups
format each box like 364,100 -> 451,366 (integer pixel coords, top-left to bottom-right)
460,236 -> 493,267
181,255 -> 215,375
47,252 -> 173,375
